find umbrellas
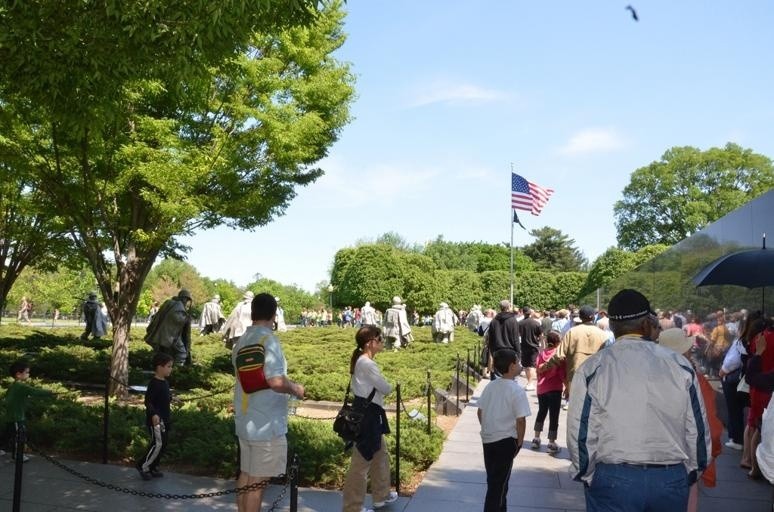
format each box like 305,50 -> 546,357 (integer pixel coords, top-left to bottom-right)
685,232 -> 774,335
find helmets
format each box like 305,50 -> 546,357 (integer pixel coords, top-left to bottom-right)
87,290 -> 97,300
243,291 -> 254,297
440,300 -> 449,308
179,287 -> 191,300
275,296 -> 279,304
211,292 -> 218,297
392,295 -> 403,306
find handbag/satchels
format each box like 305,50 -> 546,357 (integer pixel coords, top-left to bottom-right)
332,371 -> 380,444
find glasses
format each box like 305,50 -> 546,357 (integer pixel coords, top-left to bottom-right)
370,334 -> 383,341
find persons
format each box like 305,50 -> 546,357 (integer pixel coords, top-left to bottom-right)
147,302 -> 158,324
81,294 -> 107,339
480,308 -> 497,379
466,305 -> 484,331
518,306 -> 543,391
342,327 -> 398,512
360,301 -> 377,327
337,306 -> 361,328
659,327 -> 722,512
4,360 -> 52,462
533,304 -> 580,334
597,310 -> 660,354
725,311 -> 745,336
301,304 -> 332,328
719,320 -> 752,450
199,295 -> 226,337
531,332 -> 572,453
745,334 -> 774,478
221,291 -> 253,350
741,316 -> 774,469
516,308 -> 525,371
536,305 -> 613,461
383,296 -> 414,350
596,311 -> 610,330
655,308 -> 730,378
458,309 -> 468,326
272,297 -> 287,333
568,288 -> 714,512
135,353 -> 183,480
18,296 -> 30,323
101,301 -> 108,316
487,300 -> 521,381
232,294 -> 304,512
53,309 -> 59,322
145,290 -> 193,369
477,349 -> 532,512
413,310 -> 433,327
432,303 -> 459,343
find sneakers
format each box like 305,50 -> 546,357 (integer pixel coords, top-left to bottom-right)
134,459 -> 150,483
722,436 -> 742,449
147,460 -> 165,480
530,434 -> 539,450
544,440 -> 562,455
11,451 -> 32,463
375,489 -> 402,509
358,506 -> 375,511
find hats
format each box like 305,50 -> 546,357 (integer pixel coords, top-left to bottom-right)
576,303 -> 599,325
606,287 -> 661,322
656,327 -> 696,355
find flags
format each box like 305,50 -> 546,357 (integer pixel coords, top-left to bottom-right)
513,209 -> 526,231
512,172 -> 554,216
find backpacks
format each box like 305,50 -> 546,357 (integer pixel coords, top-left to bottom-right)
236,336 -> 269,413
478,324 -> 488,336
